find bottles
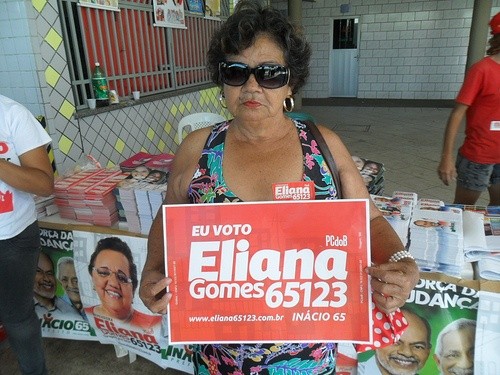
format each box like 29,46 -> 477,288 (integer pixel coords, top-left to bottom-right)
92,62 -> 110,107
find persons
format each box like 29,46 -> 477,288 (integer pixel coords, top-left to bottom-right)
435,318 -> 476,375
357,305 -> 433,375
34,250 -> 89,336
0,94 -> 54,375
437,12 -> 500,206
139,8 -> 419,375
352,155 -> 382,187
374,197 -> 402,205
413,219 -> 456,235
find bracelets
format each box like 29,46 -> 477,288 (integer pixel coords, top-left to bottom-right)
388,250 -> 417,264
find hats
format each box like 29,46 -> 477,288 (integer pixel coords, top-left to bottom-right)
489,12 -> 500,35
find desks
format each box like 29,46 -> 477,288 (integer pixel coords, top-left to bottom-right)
35,206 -> 500,375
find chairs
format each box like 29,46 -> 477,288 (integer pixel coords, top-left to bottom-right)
178,112 -> 226,145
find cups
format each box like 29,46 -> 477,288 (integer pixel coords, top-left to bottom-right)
87,99 -> 96,110
132,90 -> 141,100
110,90 -> 119,104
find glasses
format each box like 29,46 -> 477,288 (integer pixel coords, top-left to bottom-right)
92,267 -> 133,284
218,61 -> 291,89
